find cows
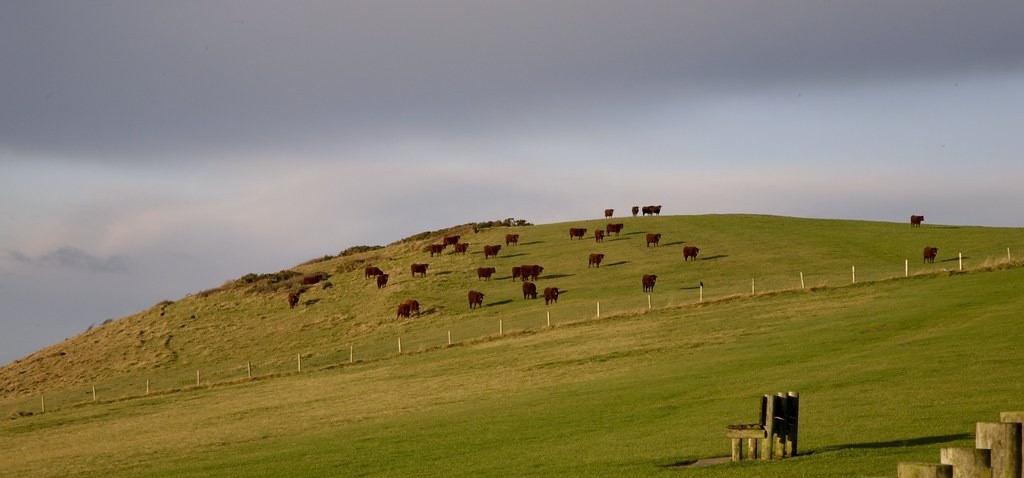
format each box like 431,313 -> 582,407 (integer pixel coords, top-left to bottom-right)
522,281 -> 537,299
364,267 -> 383,279
443,234 -> 520,260
429,243 -> 447,257
544,287 -> 558,305
646,233 -> 662,247
411,263 -> 429,277
287,293 -> 300,309
642,205 -> 662,216
468,290 -> 485,309
569,206 -> 639,242
683,246 -> 700,262
397,299 -> 419,318
642,274 -> 658,293
511,265 -> 544,281
477,267 -> 496,281
923,247 -> 939,263
588,253 -> 605,268
910,215 -> 924,228
377,273 -> 389,289
